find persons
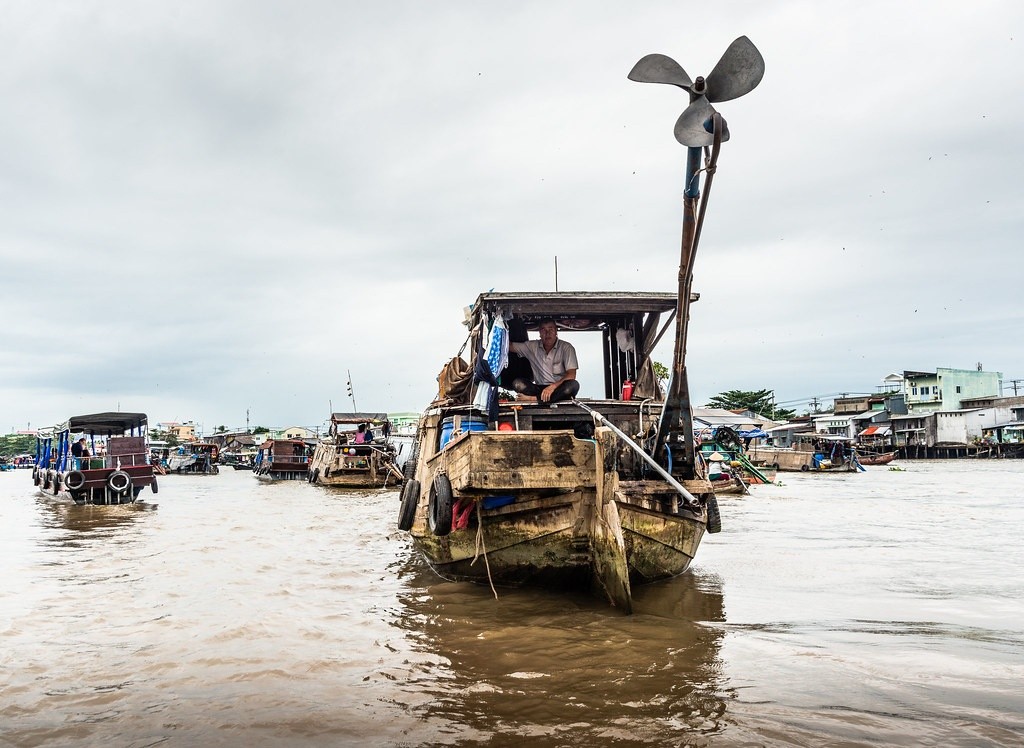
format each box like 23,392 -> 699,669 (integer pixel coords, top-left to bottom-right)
355,424 -> 373,444
706,452 -> 731,481
50,448 -> 58,459
71,438 -> 86,457
508,318 -> 579,405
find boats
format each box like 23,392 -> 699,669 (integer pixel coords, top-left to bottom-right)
182,441 -> 219,464
232,452 -> 259,470
307,413 -> 405,488
851,446 -> 900,465
252,439 -> 313,481
791,432 -> 859,473
693,425 -> 777,484
32,412 -> 158,506
697,449 -> 751,495
0,456 -> 8,471
224,451 -> 235,466
14,454 -> 36,469
398,35 -> 765,615
7,457 -> 15,469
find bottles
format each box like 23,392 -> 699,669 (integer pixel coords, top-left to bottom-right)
622,378 -> 632,401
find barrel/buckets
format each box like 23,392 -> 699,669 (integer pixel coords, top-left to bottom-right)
440,416 -> 488,451
815,452 -> 824,468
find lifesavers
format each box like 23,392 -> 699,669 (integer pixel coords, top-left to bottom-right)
869,455 -> 875,463
306,468 -> 320,483
32,464 -> 63,496
397,459 -> 421,531
324,467 -> 330,478
306,448 -> 313,456
701,493 -> 722,534
252,464 -> 271,476
772,462 -> 780,472
801,464 -> 808,472
426,473 -> 453,537
150,473 -> 159,495
64,471 -> 86,491
105,471 -> 132,493
